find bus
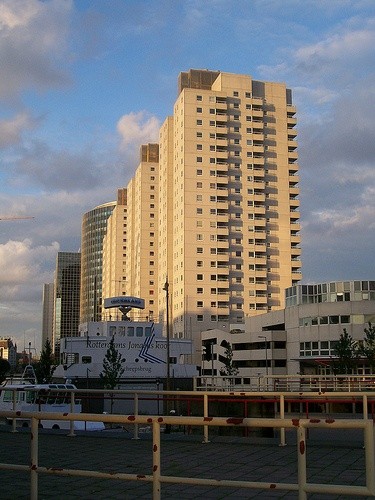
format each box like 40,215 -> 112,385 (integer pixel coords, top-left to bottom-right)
0,383 -> 104,432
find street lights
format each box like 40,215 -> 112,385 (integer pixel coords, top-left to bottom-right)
258,335 -> 269,391
155,377 -> 161,416
